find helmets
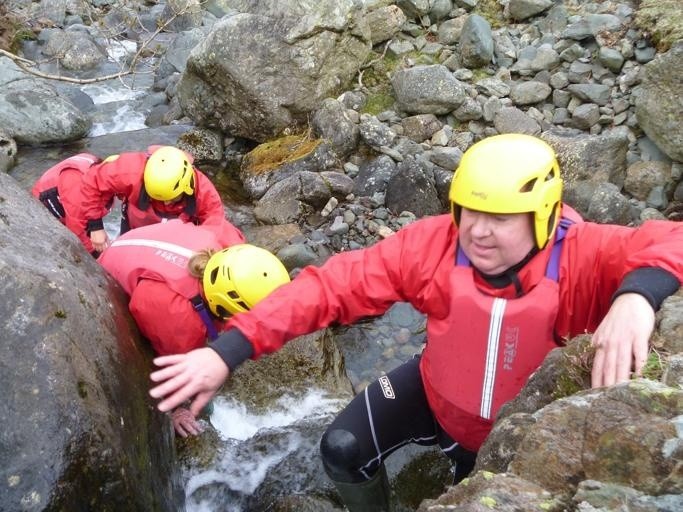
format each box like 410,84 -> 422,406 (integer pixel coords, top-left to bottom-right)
143,145 -> 195,202
203,243 -> 294,323
447,132 -> 564,250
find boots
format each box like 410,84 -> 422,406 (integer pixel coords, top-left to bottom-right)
321,464 -> 393,511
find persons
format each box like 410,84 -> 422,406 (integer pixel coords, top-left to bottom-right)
94,220 -> 292,438
79,142 -> 224,256
149,134 -> 682,510
30,148 -> 128,263
